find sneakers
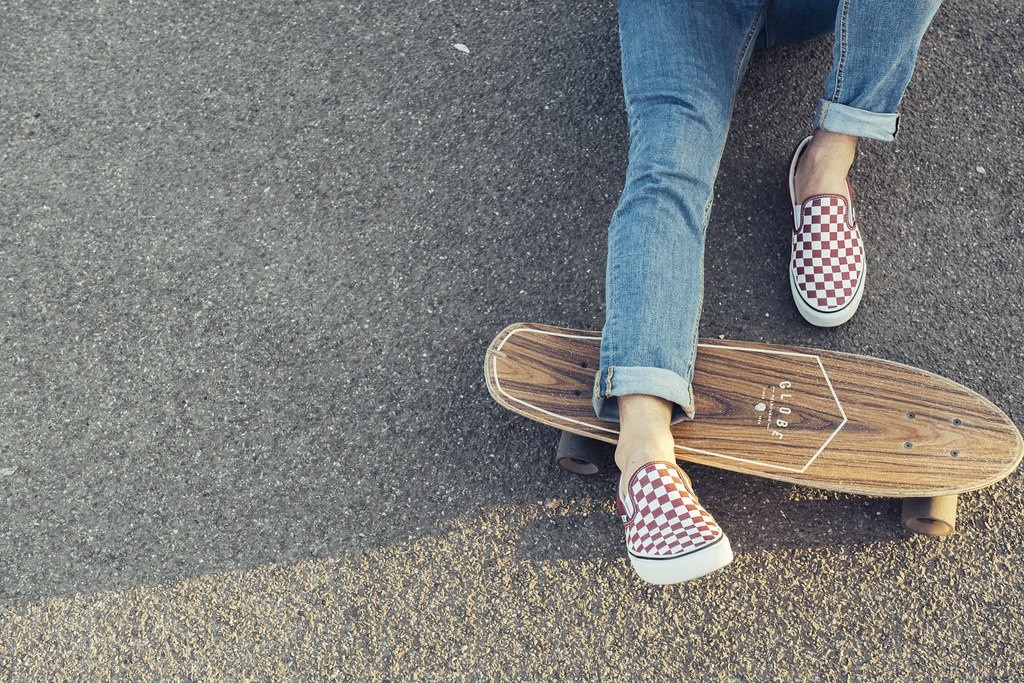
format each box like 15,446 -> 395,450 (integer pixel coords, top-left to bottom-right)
616,462 -> 734,585
788,134 -> 867,329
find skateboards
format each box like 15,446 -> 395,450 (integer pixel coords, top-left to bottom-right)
483,321 -> 1024,537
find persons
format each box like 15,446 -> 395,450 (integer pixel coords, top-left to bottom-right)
591,0 -> 943,585
